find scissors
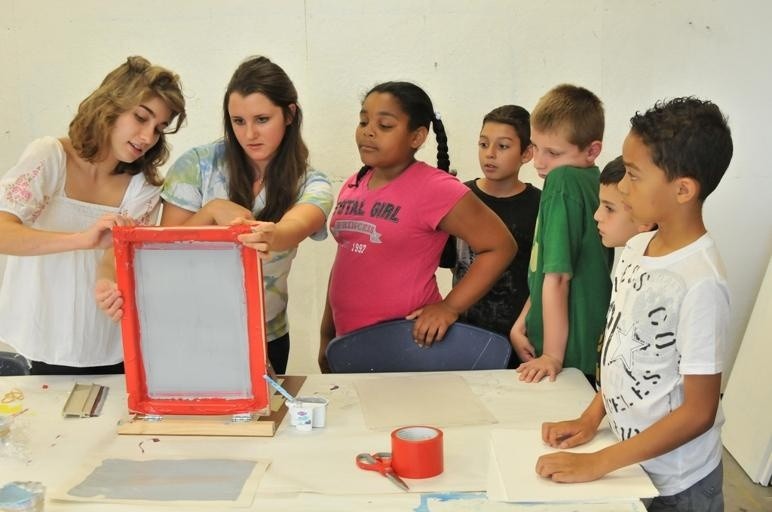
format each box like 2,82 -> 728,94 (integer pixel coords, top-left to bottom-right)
356,452 -> 409,491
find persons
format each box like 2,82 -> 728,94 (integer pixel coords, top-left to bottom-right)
593,145 -> 657,250
0,52 -> 189,374
316,80 -> 521,374
433,100 -> 545,370
534,93 -> 735,512
506,80 -> 613,393
157,53 -> 335,374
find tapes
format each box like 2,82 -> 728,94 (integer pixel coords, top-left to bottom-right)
391,426 -> 444,479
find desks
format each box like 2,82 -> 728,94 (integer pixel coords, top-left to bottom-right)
0,368 -> 651,512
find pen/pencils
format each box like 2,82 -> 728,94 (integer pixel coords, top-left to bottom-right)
263,374 -> 296,402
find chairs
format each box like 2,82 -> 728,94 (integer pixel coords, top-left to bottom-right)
323,318 -> 513,373
